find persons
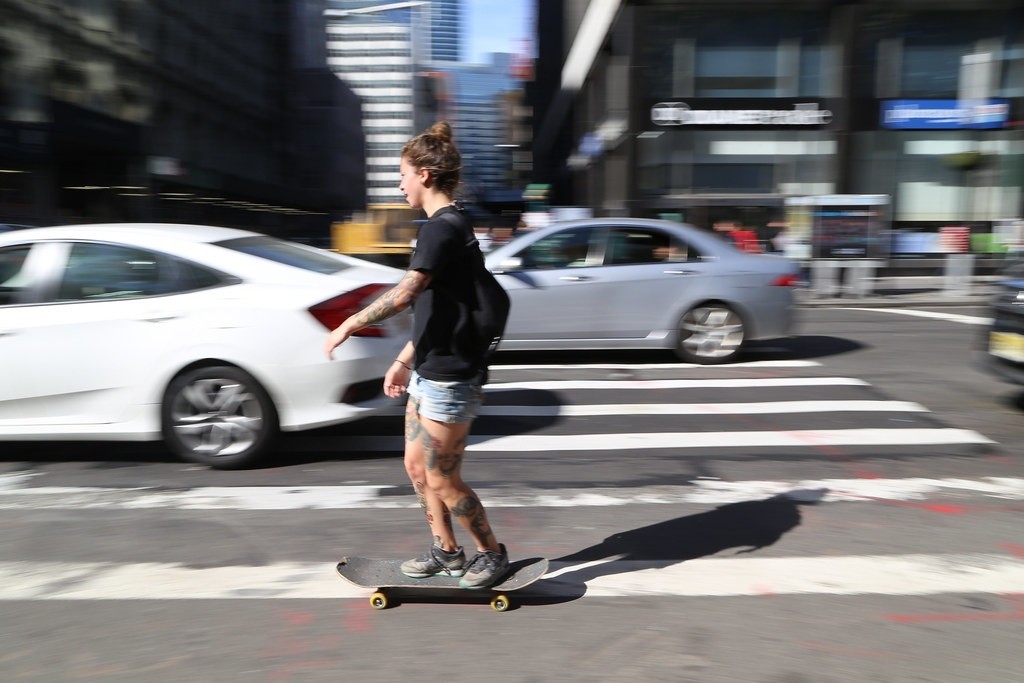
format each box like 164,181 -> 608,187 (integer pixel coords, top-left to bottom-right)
652,215 -> 799,268
325,119 -> 513,590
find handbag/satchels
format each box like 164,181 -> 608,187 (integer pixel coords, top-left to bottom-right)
429,210 -> 511,363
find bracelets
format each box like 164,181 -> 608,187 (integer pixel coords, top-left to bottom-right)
393,359 -> 412,371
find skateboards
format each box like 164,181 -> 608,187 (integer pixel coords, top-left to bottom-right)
336,556 -> 549,612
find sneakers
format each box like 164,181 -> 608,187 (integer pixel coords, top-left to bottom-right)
457,543 -> 511,589
402,546 -> 469,579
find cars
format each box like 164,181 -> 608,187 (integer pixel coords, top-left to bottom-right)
978,279 -> 1024,386
0,221 -> 415,468
482,215 -> 804,367
